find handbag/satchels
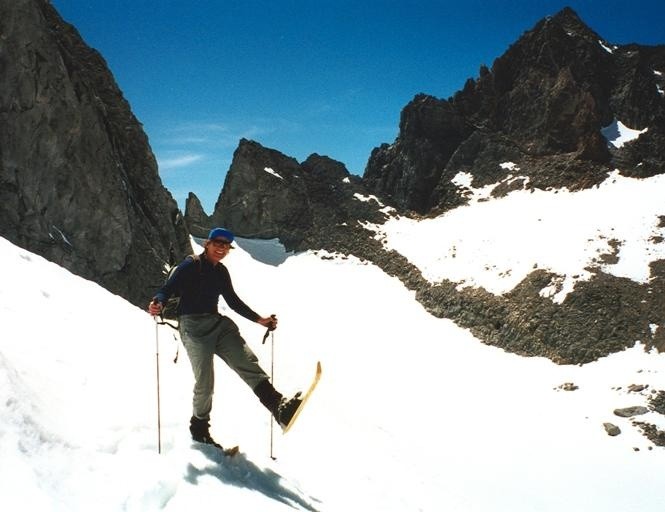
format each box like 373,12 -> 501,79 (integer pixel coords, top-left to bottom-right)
159,254 -> 201,319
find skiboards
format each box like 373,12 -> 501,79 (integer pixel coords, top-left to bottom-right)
191,361 -> 321,457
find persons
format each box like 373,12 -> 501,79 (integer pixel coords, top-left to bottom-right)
149,228 -> 303,449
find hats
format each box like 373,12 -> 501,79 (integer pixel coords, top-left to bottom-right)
208,228 -> 234,242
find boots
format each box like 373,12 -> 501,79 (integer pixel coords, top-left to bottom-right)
189,415 -> 221,450
254,381 -> 303,428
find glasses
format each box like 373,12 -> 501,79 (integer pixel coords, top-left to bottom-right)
209,238 -> 231,250
